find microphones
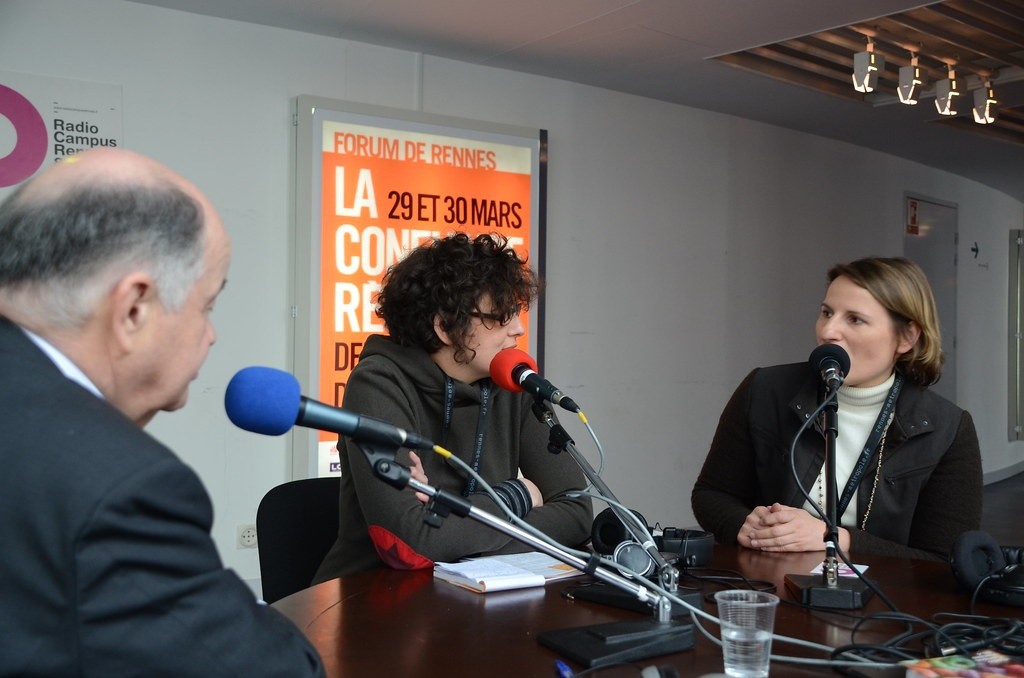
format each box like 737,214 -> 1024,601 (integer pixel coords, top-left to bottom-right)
810,344 -> 851,389
490,348 -> 580,413
224,367 -> 434,450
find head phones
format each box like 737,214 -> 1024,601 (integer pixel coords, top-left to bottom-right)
950,529 -> 1024,606
591,506 -> 715,567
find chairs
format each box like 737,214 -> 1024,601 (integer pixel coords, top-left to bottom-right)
257,476 -> 340,604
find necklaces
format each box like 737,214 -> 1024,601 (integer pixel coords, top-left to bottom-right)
814,410 -> 894,528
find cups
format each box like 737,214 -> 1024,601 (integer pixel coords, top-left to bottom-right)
713,589 -> 780,677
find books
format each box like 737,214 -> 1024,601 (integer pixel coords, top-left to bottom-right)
431,552 -> 586,592
897,650 -> 1024,678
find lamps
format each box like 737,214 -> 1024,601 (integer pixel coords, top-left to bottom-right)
935,63 -> 966,115
973,76 -> 1001,124
852,36 -> 885,92
897,50 -> 928,104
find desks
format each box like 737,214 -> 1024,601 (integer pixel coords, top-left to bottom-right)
268,542 -> 1024,678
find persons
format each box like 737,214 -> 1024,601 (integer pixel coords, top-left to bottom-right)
0,143 -> 328,678
693,254 -> 984,566
336,230 -> 595,572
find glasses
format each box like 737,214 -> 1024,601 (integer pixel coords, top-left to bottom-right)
466,302 -> 522,327
